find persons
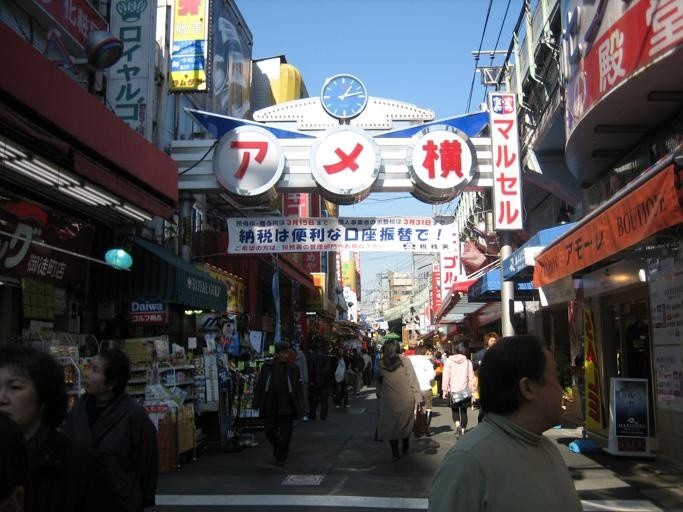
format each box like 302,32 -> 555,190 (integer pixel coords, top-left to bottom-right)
1,337 -> 143,511
0,412 -> 28,512
68,348 -> 161,511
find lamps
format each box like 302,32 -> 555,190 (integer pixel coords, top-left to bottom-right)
103,222 -> 135,272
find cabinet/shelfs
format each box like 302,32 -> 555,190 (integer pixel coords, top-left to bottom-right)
9,331 -> 194,415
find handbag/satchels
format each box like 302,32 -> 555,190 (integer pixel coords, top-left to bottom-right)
412,402 -> 433,436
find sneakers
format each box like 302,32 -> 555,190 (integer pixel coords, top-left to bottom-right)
453,421 -> 465,439
390,444 -> 410,463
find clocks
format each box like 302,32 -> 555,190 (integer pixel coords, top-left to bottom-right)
322,74 -> 368,121
